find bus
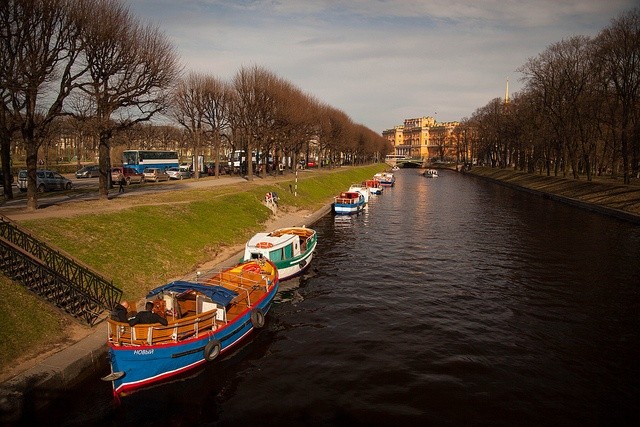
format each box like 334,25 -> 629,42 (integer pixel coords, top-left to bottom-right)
121,150 -> 180,174
225,150 -> 273,169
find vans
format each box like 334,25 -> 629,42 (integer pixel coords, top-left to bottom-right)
17,170 -> 73,192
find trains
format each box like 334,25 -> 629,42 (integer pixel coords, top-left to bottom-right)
382,155 -> 406,160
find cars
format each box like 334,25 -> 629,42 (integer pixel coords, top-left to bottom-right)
166,167 -> 191,180
75,165 -> 99,178
143,168 -> 170,183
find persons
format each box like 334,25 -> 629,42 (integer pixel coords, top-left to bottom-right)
129,301 -> 168,327
109,301 -> 128,322
117,169 -> 126,192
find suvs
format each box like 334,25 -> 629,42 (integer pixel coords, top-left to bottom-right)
111,167 -> 145,185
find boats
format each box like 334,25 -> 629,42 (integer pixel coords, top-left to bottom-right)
334,190 -> 366,215
423,168 -> 439,178
372,172 -> 395,186
239,225 -> 317,281
101,256 -> 281,403
392,166 -> 400,171
362,180 -> 385,195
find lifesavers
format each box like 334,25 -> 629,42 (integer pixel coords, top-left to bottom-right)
241,263 -> 261,278
256,241 -> 273,249
250,307 -> 265,328
204,338 -> 222,361
